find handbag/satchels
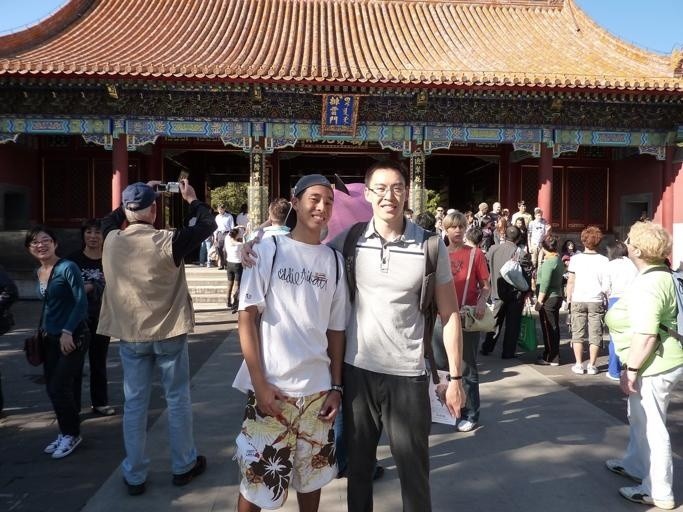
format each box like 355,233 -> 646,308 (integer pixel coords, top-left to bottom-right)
460,303 -> 497,332
25,333 -> 43,367
497,268 -> 531,303
500,259 -> 530,292
517,316 -> 537,352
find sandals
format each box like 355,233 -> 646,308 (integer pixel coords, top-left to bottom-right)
90,405 -> 116,415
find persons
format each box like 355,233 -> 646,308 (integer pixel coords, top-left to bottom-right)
230,175 -> 353,512
25,179 -> 218,494
194,198 -> 291,306
559,217 -> 682,509
239,163 -> 467,512
404,198 -> 567,432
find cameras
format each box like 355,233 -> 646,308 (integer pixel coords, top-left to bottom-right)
155,182 -> 182,194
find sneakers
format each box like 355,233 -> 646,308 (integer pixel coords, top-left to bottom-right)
605,372 -> 621,381
337,462 -> 384,481
618,484 -> 675,510
123,477 -> 145,496
587,364 -> 599,375
606,459 -> 642,484
44,433 -> 64,454
536,358 -> 560,366
457,420 -> 478,432
571,364 -> 585,374
173,456 -> 206,486
52,435 -> 82,458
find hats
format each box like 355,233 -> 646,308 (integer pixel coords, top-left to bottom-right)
123,182 -> 161,212
284,174 -> 331,229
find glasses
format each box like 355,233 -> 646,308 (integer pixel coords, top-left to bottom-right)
29,239 -> 52,247
366,186 -> 407,197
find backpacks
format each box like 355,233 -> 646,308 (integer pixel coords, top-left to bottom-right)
644,267 -> 683,350
